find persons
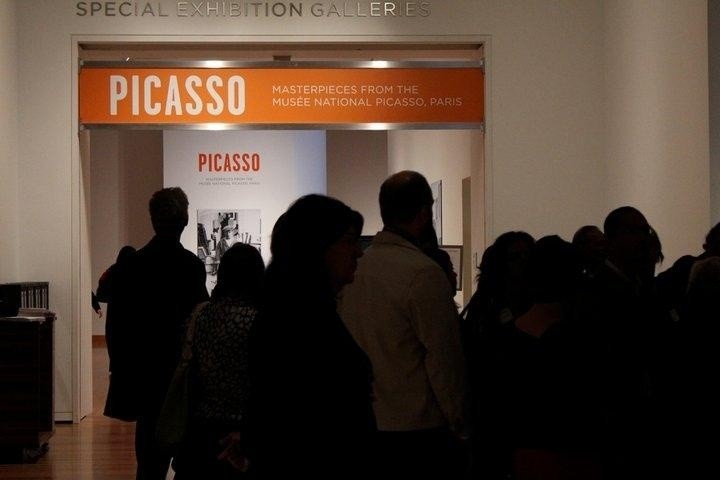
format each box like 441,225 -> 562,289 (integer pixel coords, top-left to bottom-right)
429,208 -> 719,480
97,245 -> 137,373
239,193 -> 381,479
91,292 -> 102,320
337,170 -> 471,479
111,186 -> 212,479
154,240 -> 267,479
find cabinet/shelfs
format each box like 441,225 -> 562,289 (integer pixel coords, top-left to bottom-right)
0,311 -> 56,464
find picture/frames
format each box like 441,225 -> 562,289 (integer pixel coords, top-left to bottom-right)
439,243 -> 464,292
